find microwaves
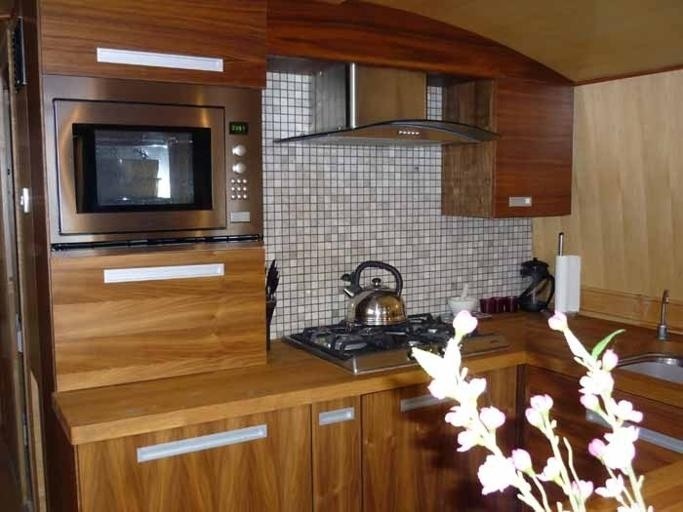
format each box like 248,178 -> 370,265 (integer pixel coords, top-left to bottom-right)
37,72 -> 262,247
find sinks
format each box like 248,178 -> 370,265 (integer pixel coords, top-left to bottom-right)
612,348 -> 683,386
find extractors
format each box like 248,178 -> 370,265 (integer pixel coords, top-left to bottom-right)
273,62 -> 499,152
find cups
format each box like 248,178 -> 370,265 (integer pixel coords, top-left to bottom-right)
447,294 -> 517,317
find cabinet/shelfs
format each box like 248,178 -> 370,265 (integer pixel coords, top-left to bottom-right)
40,389 -> 313,512
441,0 -> 574,219
34,248 -> 267,393
310,380 -> 363,512
362,353 -> 517,512
21,1 -> 268,90
526,365 -> 683,512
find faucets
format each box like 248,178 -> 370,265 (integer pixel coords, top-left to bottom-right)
656,289 -> 670,341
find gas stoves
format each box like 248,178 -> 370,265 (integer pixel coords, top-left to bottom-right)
285,310 -> 507,374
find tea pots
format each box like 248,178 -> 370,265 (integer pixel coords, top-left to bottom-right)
340,262 -> 408,326
518,259 -> 552,310
